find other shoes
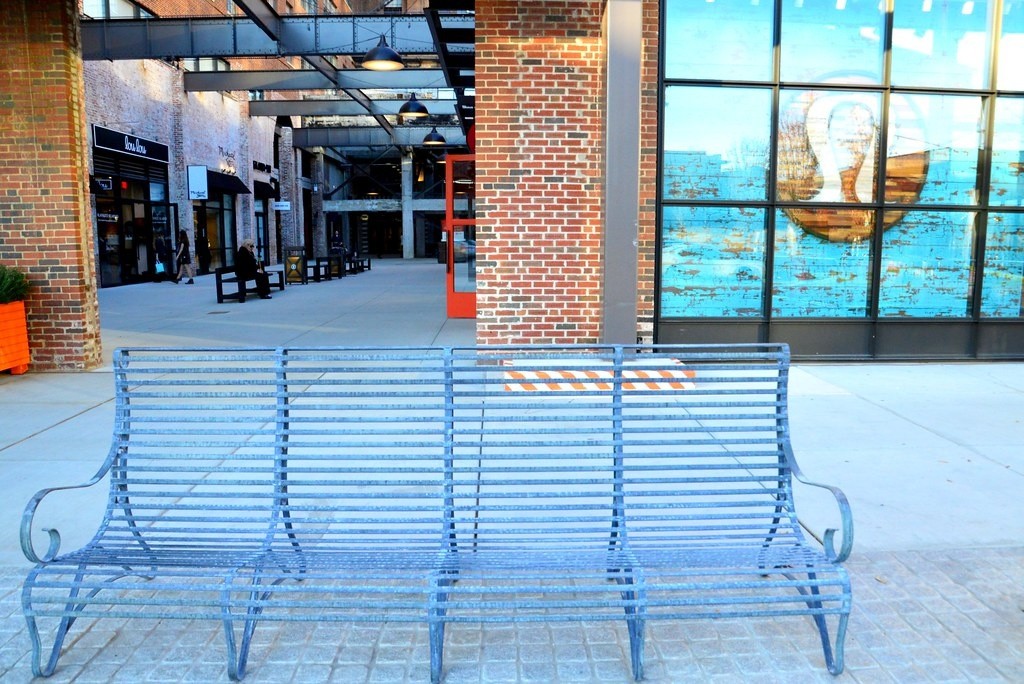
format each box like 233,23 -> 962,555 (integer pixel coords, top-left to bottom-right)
260,294 -> 272,299
185,279 -> 194,284
171,278 -> 178,284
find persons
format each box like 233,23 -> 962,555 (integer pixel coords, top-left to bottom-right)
151,232 -> 166,282
235,238 -> 272,299
171,230 -> 194,285
330,230 -> 343,256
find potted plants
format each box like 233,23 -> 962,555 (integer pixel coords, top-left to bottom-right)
0,264 -> 31,375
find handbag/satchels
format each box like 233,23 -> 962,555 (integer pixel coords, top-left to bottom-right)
155,260 -> 164,274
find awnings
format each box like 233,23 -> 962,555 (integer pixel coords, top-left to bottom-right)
254,180 -> 280,198
208,170 -> 252,197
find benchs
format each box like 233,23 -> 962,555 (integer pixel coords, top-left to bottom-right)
16,343 -> 854,684
215,251 -> 370,303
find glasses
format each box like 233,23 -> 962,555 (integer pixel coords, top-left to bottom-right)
248,244 -> 254,248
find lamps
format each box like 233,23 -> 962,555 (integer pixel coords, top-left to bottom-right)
398,92 -> 429,117
423,126 -> 446,145
436,154 -> 455,164
361,33 -> 405,71
